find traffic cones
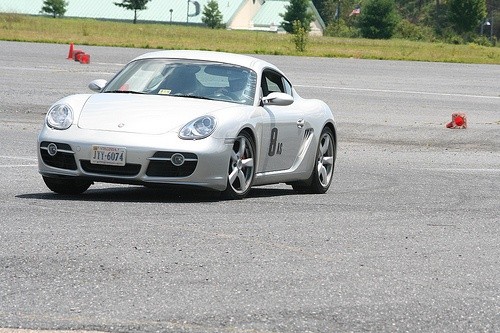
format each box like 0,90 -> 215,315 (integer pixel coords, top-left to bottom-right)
78,54 -> 90,64
446,113 -> 467,128
72,50 -> 84,61
67,42 -> 73,59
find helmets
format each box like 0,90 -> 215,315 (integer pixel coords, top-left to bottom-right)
228,72 -> 250,99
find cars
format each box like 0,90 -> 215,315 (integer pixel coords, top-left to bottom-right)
35,47 -> 337,201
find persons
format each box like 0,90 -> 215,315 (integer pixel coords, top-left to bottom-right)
161,62 -> 197,98
216,68 -> 264,106
